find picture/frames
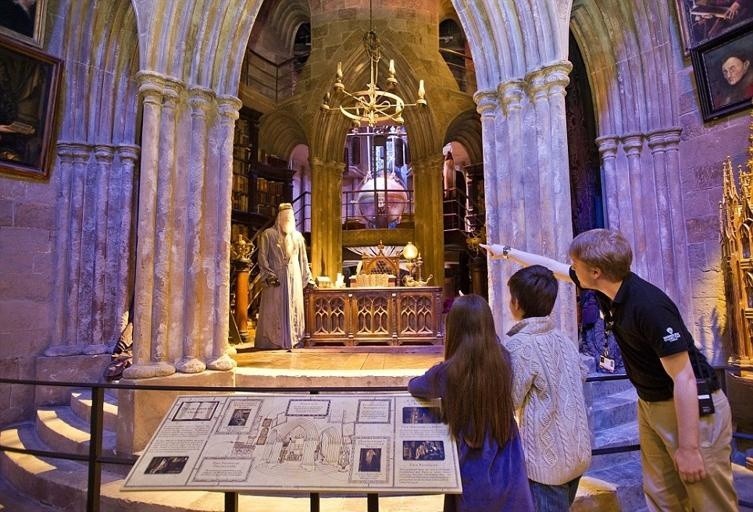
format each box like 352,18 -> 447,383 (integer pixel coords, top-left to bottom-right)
690,21 -> 753,123
0,33 -> 64,183
0,0 -> 49,50
673,0 -> 753,58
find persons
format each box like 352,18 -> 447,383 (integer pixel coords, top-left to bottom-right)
471,225 -> 753,511
252,201 -> 319,347
493,261 -> 592,510
403,289 -> 536,511
708,49 -> 751,111
697,0 -> 752,37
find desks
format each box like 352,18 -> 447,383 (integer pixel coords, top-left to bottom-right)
303,286 -> 443,346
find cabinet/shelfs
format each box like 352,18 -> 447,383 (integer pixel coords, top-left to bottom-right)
232,105 -> 294,225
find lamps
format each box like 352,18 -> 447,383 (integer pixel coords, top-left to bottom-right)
403,242 -> 418,276
320,0 -> 428,128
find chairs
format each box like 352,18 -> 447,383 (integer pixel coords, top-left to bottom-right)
361,240 -> 401,287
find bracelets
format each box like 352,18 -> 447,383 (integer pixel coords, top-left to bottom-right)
502,245 -> 511,260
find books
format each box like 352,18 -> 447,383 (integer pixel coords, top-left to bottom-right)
690,5 -> 735,19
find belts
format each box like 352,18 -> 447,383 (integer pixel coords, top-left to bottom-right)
697,379 -> 721,395
